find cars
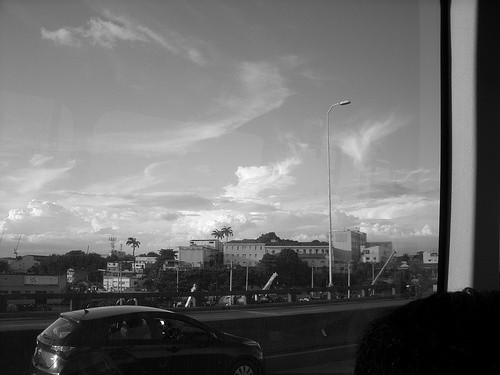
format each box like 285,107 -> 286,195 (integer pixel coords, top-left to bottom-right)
32,304 -> 264,375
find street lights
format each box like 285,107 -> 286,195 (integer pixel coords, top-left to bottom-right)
326,99 -> 351,301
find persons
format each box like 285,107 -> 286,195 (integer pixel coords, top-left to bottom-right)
409,273 -> 423,304
